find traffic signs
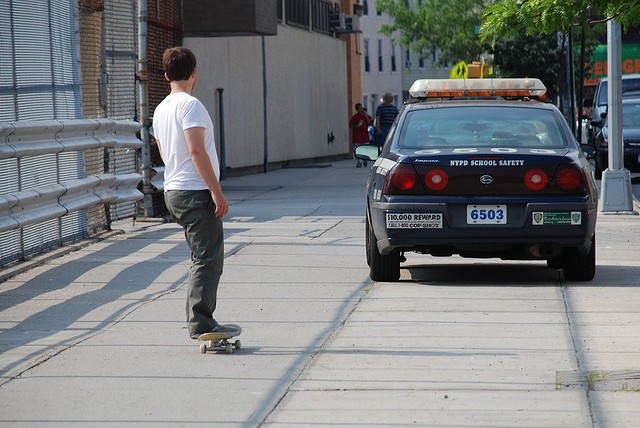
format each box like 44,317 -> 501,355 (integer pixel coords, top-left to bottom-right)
449,60 -> 468,78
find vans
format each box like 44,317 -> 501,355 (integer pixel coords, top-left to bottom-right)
586,74 -> 640,146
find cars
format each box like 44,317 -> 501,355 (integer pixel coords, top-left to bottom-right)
595,98 -> 640,180
366,78 -> 598,281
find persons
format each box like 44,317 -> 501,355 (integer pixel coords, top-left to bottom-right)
376,92 -> 399,146
350,103 -> 371,167
152,47 -> 239,339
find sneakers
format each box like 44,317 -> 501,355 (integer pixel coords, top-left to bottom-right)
357,162 -> 362,167
192,326 -> 237,338
364,162 -> 367,167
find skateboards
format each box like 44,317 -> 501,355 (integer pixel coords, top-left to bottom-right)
198,323 -> 242,354
